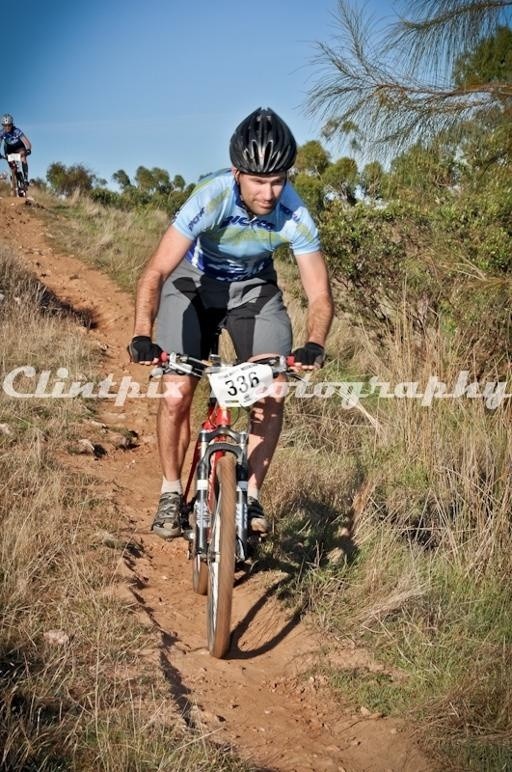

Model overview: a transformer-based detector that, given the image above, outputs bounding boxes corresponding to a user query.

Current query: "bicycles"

[150,352,328,659]
[0,149,32,199]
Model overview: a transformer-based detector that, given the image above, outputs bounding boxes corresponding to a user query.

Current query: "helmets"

[229,107,297,177]
[1,114,13,126]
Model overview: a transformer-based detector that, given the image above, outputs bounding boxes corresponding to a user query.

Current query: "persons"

[0,113,33,196]
[129,107,334,541]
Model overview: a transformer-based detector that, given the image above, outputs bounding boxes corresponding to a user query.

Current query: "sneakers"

[246,495,268,533]
[152,484,183,539]
